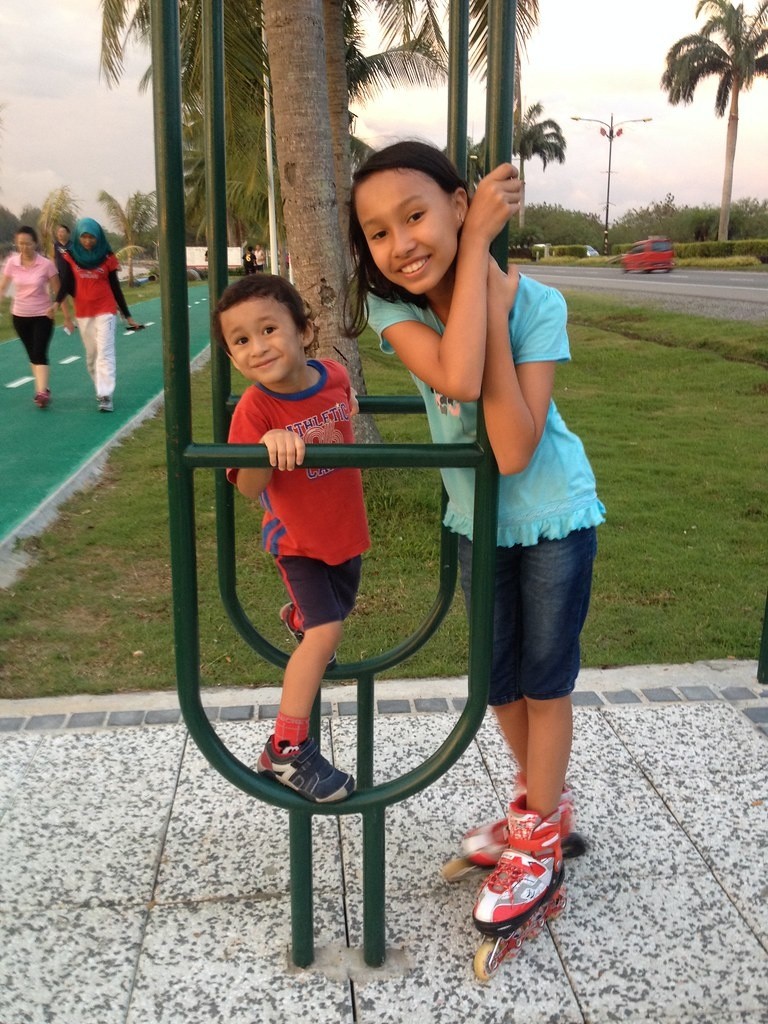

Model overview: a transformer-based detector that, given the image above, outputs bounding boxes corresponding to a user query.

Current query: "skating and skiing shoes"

[472,794,569,981]
[441,773,587,884]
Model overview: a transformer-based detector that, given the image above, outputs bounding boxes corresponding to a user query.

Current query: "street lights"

[571,113,652,256]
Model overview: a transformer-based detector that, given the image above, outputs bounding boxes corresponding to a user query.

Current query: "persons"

[212,274,371,802]
[242,246,257,275]
[254,243,266,275]
[286,253,289,269]
[336,141,606,979]
[0,226,74,408]
[46,218,140,412]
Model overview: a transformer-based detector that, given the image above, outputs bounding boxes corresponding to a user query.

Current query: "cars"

[586,246,599,257]
[621,239,676,274]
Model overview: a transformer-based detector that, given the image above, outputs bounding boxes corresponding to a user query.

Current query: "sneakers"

[96,395,101,402]
[98,396,113,411]
[257,734,355,804]
[279,602,337,672]
[33,388,50,408]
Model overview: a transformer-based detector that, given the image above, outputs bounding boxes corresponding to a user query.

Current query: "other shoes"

[64,321,74,334]
